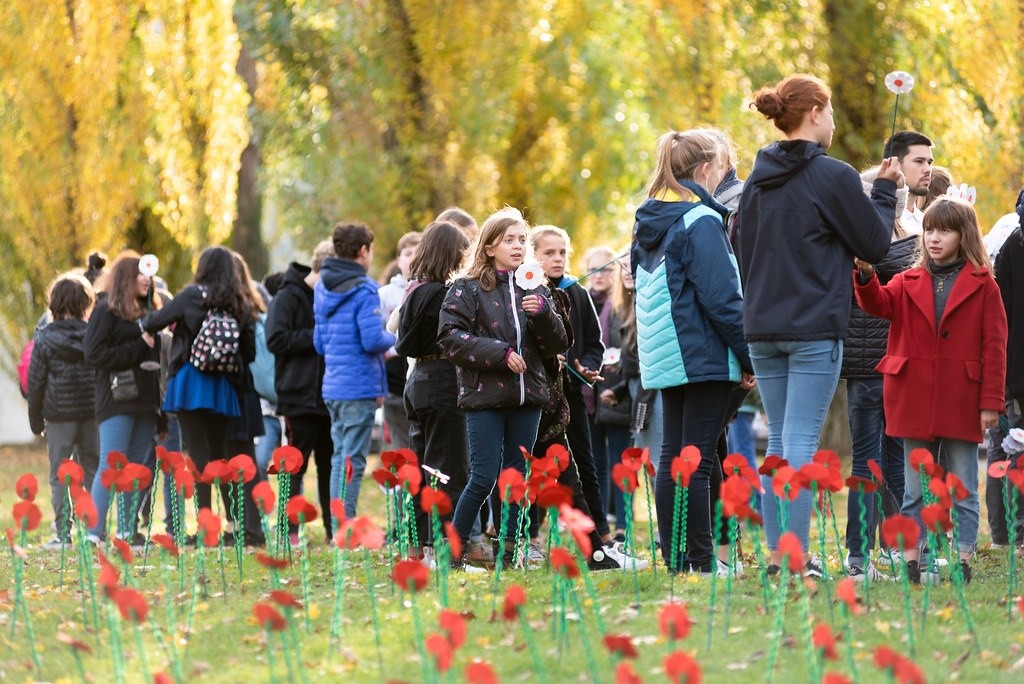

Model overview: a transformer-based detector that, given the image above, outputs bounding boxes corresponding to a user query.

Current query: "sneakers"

[919,556,948,571]
[587,546,649,573]
[846,562,890,582]
[766,565,785,578]
[878,547,909,564]
[894,565,940,585]
[790,556,833,582]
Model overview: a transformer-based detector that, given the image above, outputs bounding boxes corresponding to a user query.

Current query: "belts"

[414,353,452,363]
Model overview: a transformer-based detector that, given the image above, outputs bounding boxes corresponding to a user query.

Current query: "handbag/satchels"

[110,369,139,401]
[536,375,570,444]
[594,383,632,428]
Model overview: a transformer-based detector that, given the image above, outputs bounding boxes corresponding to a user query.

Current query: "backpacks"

[189,283,242,372]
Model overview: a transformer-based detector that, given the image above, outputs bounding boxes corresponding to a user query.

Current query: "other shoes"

[76,536,100,547]
[116,530,153,553]
[44,535,72,549]
[948,558,972,584]
[904,560,921,585]
[171,515,743,578]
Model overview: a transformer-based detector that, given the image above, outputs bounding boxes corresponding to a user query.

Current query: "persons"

[313,221,397,540]
[728,79,905,585]
[142,246,263,550]
[378,208,648,576]
[264,238,340,546]
[250,281,282,475]
[29,250,175,549]
[629,128,1024,583]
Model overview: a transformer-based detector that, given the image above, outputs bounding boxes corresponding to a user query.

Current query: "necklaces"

[933,270,958,293]
